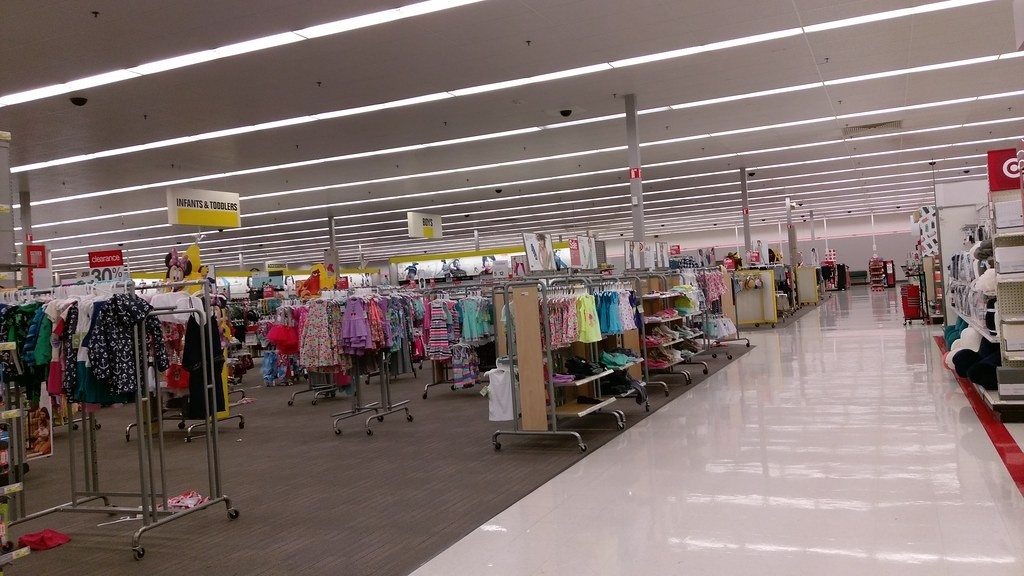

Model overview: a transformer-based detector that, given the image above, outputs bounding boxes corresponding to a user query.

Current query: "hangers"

[0,265,788,377]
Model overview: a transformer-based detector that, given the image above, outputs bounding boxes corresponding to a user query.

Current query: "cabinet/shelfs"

[491,274,669,452]
[489,285,516,357]
[631,272,719,393]
[900,148,1024,423]
[820,260,852,291]
[868,260,886,290]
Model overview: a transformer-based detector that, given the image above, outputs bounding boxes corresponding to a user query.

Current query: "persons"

[198,266,215,293]
[436,260,451,277]
[698,248,714,267]
[581,238,594,268]
[360,273,370,287]
[536,234,552,270]
[31,407,51,454]
[349,275,352,285]
[448,259,467,276]
[629,241,634,269]
[812,248,817,265]
[284,276,295,290]
[659,244,665,267]
[479,256,496,275]
[404,261,418,280]
[262,279,274,288]
[755,240,765,265]
[638,244,644,270]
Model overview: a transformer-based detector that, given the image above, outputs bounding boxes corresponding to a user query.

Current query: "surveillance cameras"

[620,203,901,239]
[219,249,222,252]
[963,171,969,174]
[118,244,124,247]
[177,242,182,244]
[929,162,936,165]
[495,189,502,193]
[324,249,328,251]
[219,229,224,232]
[71,97,88,106]
[747,173,756,176]
[465,215,469,217]
[560,110,572,117]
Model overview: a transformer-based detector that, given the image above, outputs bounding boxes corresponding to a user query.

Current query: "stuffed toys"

[161,247,192,291]
[943,296,1000,390]
